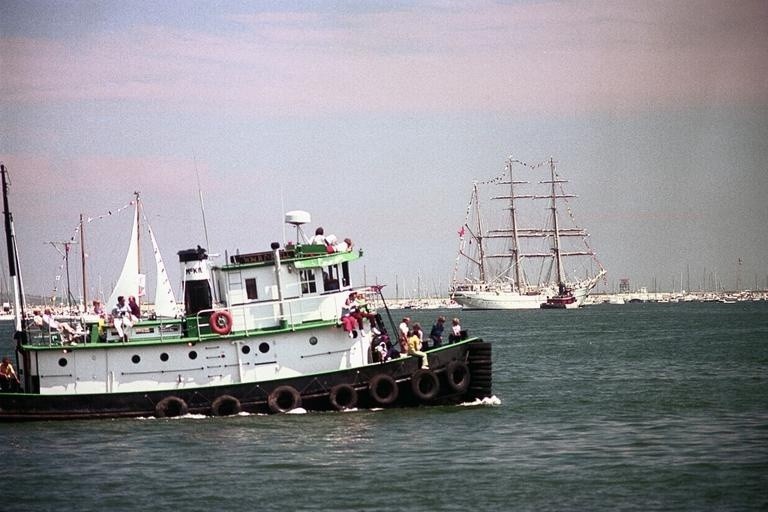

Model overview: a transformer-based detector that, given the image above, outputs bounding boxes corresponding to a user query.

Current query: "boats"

[1,160,492,418]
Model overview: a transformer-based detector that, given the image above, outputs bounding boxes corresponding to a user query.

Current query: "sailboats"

[448,156,607,309]
[657,264,768,303]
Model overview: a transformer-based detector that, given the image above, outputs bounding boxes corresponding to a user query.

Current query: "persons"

[449,316,462,344]
[312,227,325,246]
[32,308,79,347]
[90,299,107,343]
[411,322,423,352]
[1,355,22,386]
[398,315,412,355]
[341,291,381,339]
[324,239,353,254]
[43,306,77,347]
[126,296,141,328]
[111,294,132,342]
[407,329,430,372]
[0,364,19,392]
[383,340,401,363]
[428,315,446,347]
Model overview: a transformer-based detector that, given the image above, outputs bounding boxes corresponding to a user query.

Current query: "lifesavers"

[210,311,233,334]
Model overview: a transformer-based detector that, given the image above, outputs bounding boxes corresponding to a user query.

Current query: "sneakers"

[371,327,381,335]
[348,333,353,339]
[360,330,366,337]
[421,365,430,370]
